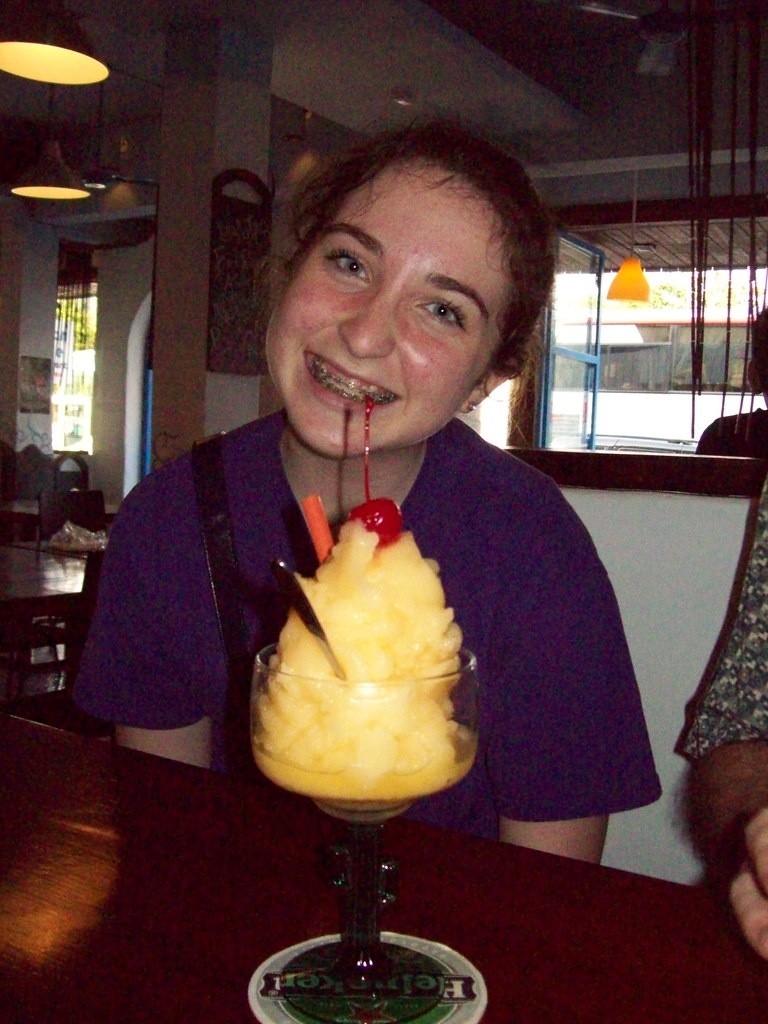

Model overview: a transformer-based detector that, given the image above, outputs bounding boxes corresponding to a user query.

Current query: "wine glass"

[242,644,481,1024]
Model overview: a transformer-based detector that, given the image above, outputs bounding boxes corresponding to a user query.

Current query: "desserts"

[251,395,474,802]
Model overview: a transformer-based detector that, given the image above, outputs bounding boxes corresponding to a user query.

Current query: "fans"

[582,0,766,78]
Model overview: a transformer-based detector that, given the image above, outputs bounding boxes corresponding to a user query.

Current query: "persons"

[81,108,666,866]
[700,304,768,459]
[677,475,768,966]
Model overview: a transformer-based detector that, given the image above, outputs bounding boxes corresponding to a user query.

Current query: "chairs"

[0,441,119,687]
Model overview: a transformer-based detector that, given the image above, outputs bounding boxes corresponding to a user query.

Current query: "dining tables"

[1,717,768,1024]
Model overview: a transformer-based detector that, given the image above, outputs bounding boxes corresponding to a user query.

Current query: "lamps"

[11,82,90,200]
[608,171,651,302]
[1,1,109,85]
